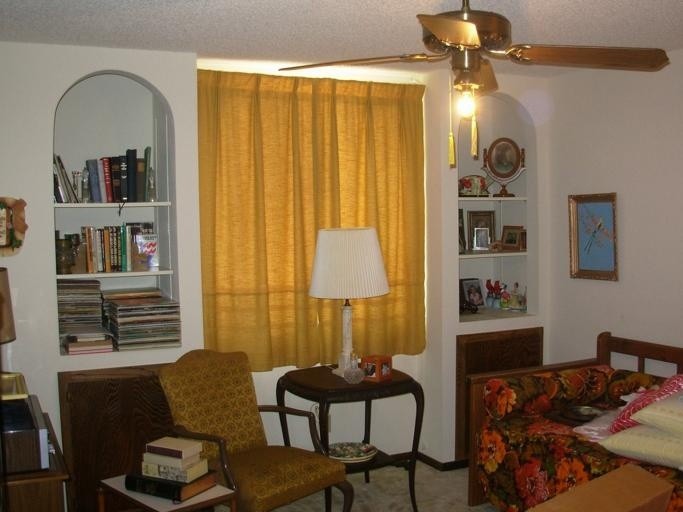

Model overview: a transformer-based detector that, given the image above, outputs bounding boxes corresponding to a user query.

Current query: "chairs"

[156,347,354,512]
[524,462,674,512]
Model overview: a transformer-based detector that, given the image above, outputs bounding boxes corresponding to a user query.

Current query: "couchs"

[463,330,682,512]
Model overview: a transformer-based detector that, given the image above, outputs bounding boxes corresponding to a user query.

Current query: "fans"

[276,1,671,95]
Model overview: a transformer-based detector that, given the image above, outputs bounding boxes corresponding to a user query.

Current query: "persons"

[469,284,483,305]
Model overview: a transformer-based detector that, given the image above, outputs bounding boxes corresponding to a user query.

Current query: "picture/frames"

[500,224,523,251]
[567,191,620,282]
[466,210,496,253]
[360,354,393,383]
[1,195,28,259]
[482,137,527,197]
[462,277,488,309]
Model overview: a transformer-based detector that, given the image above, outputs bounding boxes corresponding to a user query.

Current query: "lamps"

[447,1,486,168]
[306,225,391,377]
[0,267,17,345]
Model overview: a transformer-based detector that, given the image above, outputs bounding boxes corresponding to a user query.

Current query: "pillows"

[609,372,683,432]
[629,389,683,431]
[599,425,683,471]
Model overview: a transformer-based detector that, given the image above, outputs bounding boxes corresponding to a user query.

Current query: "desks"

[0,371,72,512]
[99,472,235,511]
[274,366,425,512]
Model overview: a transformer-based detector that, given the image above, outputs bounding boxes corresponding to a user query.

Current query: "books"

[124,436,218,502]
[53,145,182,355]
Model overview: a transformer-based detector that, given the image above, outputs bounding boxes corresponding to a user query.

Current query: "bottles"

[343,351,366,385]
[486,293,501,309]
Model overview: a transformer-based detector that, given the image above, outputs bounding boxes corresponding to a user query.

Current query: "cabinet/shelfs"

[456,192,547,465]
[56,200,197,511]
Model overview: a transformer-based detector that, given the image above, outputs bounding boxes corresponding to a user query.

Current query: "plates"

[329,442,378,464]
